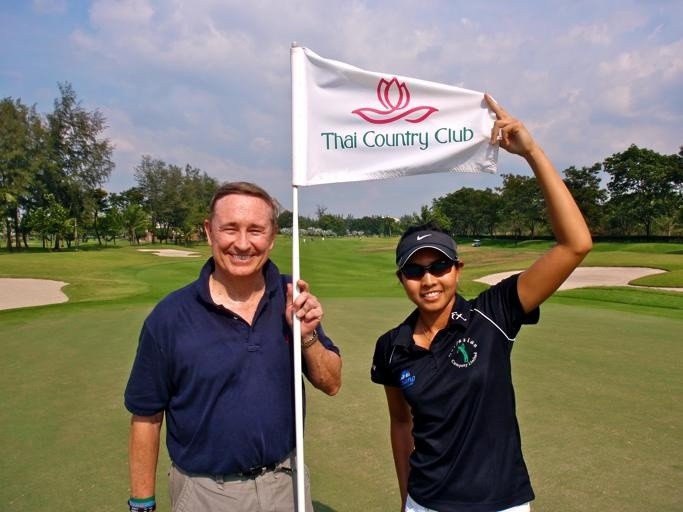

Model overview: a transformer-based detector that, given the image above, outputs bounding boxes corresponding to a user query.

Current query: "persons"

[121,181,341,512]
[370,92,591,512]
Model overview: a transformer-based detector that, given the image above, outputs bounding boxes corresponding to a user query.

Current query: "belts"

[215,463,276,482]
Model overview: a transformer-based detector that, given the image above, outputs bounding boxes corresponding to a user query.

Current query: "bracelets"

[127,499,154,507]
[129,494,154,503]
[129,505,155,512]
[302,330,318,348]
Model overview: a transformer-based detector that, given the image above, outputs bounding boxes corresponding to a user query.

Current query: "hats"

[396,230,457,269]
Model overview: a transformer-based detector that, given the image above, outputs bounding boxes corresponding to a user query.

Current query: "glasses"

[401,259,453,279]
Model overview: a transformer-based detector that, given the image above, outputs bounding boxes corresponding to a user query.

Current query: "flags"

[289,46,497,186]
